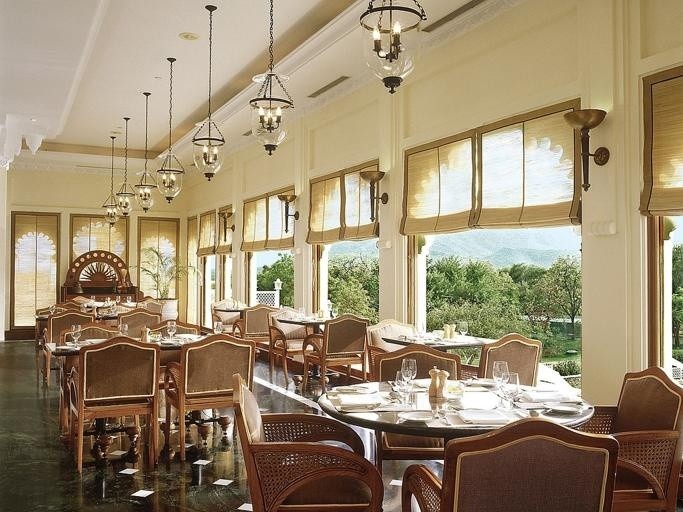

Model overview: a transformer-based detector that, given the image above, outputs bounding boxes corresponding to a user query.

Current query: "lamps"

[356,168,390,222]
[101,52,184,228]
[563,107,613,191]
[216,211,235,243]
[245,0,295,158]
[358,0,429,94]
[191,1,226,184]
[277,192,302,234]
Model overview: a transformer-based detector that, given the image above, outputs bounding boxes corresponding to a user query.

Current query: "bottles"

[441,323,455,340]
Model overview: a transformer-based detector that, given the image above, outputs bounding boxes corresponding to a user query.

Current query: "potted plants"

[129,244,198,320]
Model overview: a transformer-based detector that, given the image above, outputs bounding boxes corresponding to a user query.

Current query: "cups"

[415,321,424,338]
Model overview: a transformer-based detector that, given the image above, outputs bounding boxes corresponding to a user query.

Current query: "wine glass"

[48,305,55,315]
[395,360,519,408]
[70,320,222,349]
[459,322,467,338]
[90,295,132,317]
[287,302,337,320]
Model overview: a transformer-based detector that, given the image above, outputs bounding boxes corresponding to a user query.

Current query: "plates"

[332,378,584,423]
[55,333,204,349]
